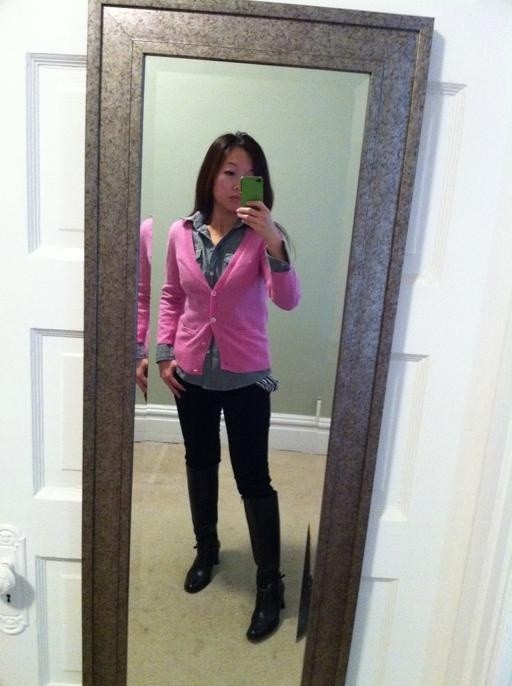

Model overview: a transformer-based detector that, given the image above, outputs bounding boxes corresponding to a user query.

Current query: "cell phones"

[240,176,263,207]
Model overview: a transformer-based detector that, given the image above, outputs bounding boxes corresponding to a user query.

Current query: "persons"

[136,215,155,404]
[158,131,303,645]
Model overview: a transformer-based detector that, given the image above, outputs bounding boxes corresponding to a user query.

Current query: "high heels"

[246,581,286,642]
[184,542,221,593]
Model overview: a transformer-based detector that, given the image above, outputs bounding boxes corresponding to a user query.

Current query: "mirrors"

[81,0,437,684]
